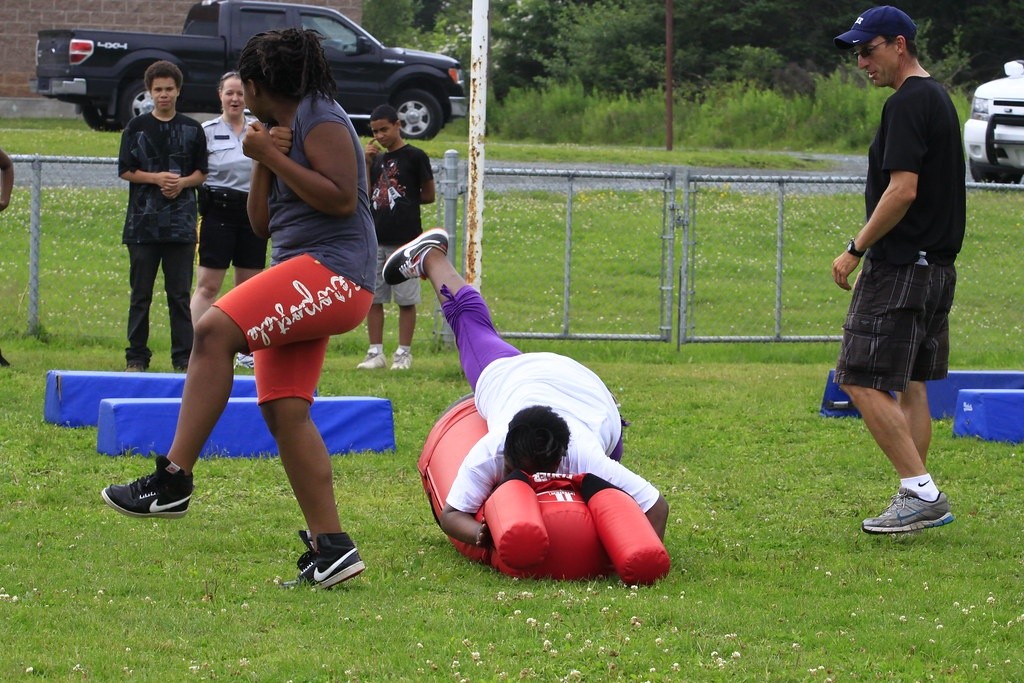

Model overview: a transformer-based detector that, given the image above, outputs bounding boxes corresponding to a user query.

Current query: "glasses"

[854,39,888,56]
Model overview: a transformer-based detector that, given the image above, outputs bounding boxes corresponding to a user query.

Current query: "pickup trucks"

[33,0,466,142]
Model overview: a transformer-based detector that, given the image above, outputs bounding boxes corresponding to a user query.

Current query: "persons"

[191,72,266,369]
[355,104,436,369]
[118,60,210,374]
[0,149,14,369]
[831,5,966,534]
[102,28,380,590]
[381,227,669,548]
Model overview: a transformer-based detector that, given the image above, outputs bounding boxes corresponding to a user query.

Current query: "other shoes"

[174,365,188,373]
[123,363,145,373]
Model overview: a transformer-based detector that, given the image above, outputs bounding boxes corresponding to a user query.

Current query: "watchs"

[848,240,866,258]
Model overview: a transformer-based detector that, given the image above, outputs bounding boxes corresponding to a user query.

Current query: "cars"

[962,60,1024,186]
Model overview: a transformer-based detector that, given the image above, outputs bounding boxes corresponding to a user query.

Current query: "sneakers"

[102,456,194,518]
[390,349,413,371]
[357,347,388,370]
[280,529,365,591]
[381,227,448,286]
[862,487,954,535]
[233,354,254,370]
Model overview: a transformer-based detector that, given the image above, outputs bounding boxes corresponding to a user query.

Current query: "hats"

[833,5,918,50]
